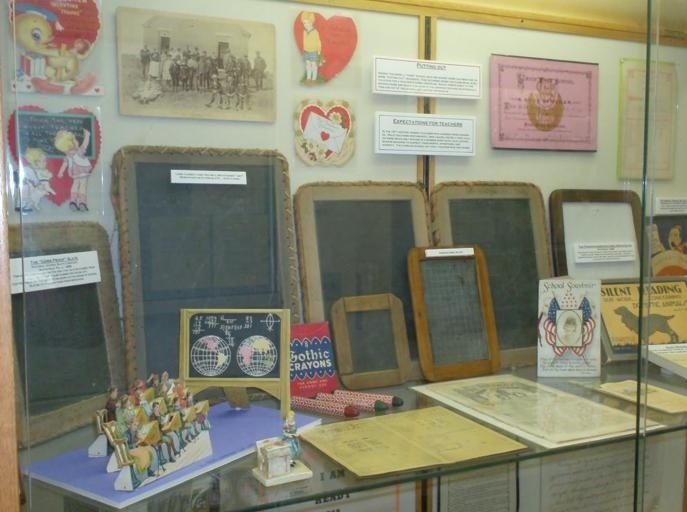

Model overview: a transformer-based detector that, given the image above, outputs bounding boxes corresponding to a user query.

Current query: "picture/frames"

[405,244,501,383]
[113,5,279,124]
[546,186,643,280]
[426,178,556,370]
[108,142,303,409]
[405,371,670,452]
[177,306,293,420]
[329,292,414,391]
[598,274,687,366]
[292,179,436,383]
[10,219,129,452]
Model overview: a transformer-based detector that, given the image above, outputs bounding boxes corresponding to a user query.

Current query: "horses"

[204,65,253,112]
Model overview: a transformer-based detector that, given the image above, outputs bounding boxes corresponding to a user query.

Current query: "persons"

[142,41,264,112]
